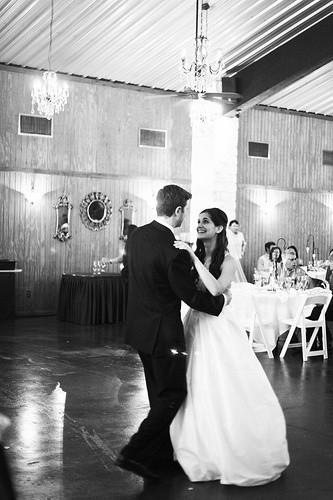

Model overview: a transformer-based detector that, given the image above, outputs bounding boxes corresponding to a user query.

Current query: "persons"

[173,208,290,487]
[102,224,137,264]
[257,240,333,290]
[114,185,232,500]
[226,219,247,260]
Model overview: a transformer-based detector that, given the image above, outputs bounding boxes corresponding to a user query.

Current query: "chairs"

[242,295,275,358]
[279,295,333,361]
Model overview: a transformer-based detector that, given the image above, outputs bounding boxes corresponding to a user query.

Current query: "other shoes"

[115,454,161,481]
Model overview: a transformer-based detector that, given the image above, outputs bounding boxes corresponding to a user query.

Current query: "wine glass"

[308,260,330,271]
[255,272,307,290]
[93,260,106,275]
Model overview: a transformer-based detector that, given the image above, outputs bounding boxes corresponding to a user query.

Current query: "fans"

[141,0,243,107]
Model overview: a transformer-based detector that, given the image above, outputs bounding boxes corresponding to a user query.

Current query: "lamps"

[180,0,223,78]
[31,0,69,120]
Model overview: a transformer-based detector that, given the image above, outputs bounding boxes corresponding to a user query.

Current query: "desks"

[232,282,332,353]
[56,273,128,326]
